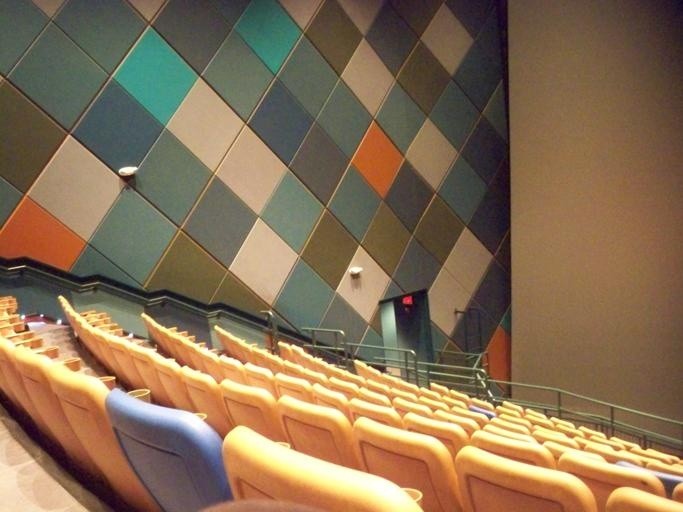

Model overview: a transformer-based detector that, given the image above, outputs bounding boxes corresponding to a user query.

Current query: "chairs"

[449,389,469,404]
[430,382,449,396]
[366,366,381,382]
[454,445,598,512]
[244,362,274,398]
[483,423,540,443]
[645,461,683,476]
[353,359,367,379]
[154,323,167,348]
[301,353,312,369]
[141,313,152,333]
[579,426,607,440]
[107,336,146,389]
[532,424,566,437]
[215,326,230,352]
[418,395,450,412]
[128,343,174,407]
[329,376,358,400]
[58,296,96,329]
[525,409,548,421]
[153,356,197,413]
[496,406,521,418]
[63,303,107,346]
[590,436,625,451]
[433,408,480,438]
[532,429,580,449]
[200,350,220,383]
[171,334,193,367]
[380,373,396,387]
[402,412,469,460]
[366,379,390,397]
[274,373,311,403]
[228,334,245,358]
[550,417,575,429]
[312,359,327,374]
[399,381,420,397]
[81,323,114,373]
[311,382,350,425]
[442,395,468,409]
[503,401,524,413]
[284,360,304,378]
[672,482,683,502]
[490,416,532,436]
[498,413,532,431]
[14,343,107,482]
[49,359,164,512]
[557,451,667,512]
[94,329,131,385]
[251,347,268,366]
[541,441,608,463]
[574,436,613,450]
[239,342,253,361]
[525,414,555,431]
[69,312,97,357]
[278,341,290,359]
[327,364,342,377]
[556,424,585,437]
[615,460,683,498]
[351,416,463,512]
[303,368,328,385]
[266,353,283,372]
[468,404,495,420]
[344,371,364,388]
[218,378,286,443]
[533,425,567,437]
[630,447,672,465]
[604,485,683,512]
[391,397,434,417]
[646,448,680,464]
[162,328,183,362]
[471,397,495,410]
[105,385,232,512]
[291,345,303,362]
[0,296,59,348]
[348,398,402,428]
[222,425,426,512]
[358,387,392,406]
[180,364,233,438]
[390,389,417,404]
[583,444,643,466]
[220,356,242,384]
[183,342,205,370]
[469,430,557,473]
[419,386,441,401]
[610,437,641,451]
[275,395,360,471]
[450,406,489,428]
